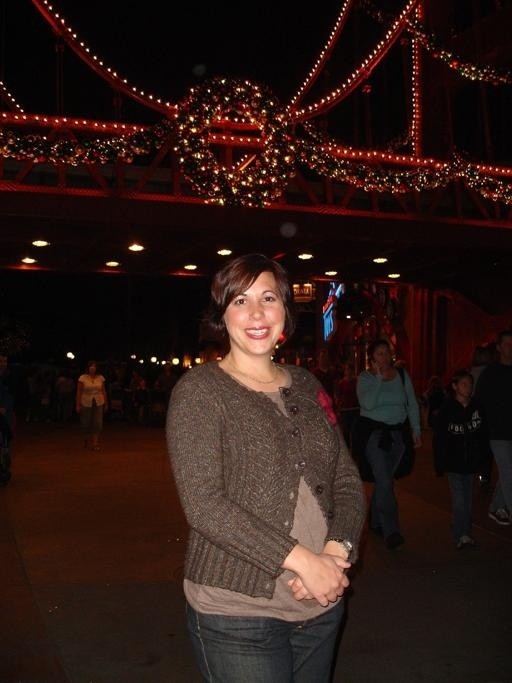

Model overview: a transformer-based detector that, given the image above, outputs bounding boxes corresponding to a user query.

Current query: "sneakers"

[456,535,481,559]
[487,508,512,526]
[367,527,407,549]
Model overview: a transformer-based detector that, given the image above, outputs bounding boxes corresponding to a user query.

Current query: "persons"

[25,353,180,428]
[334,362,360,447]
[432,370,494,550]
[475,327,512,525]
[354,335,423,552]
[309,346,343,400]
[161,251,369,683]
[0,352,16,453]
[416,365,458,479]
[74,359,110,451]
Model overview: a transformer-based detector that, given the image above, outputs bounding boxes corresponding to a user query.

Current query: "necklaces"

[227,358,280,384]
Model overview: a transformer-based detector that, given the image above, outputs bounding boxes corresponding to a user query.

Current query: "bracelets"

[337,538,354,558]
